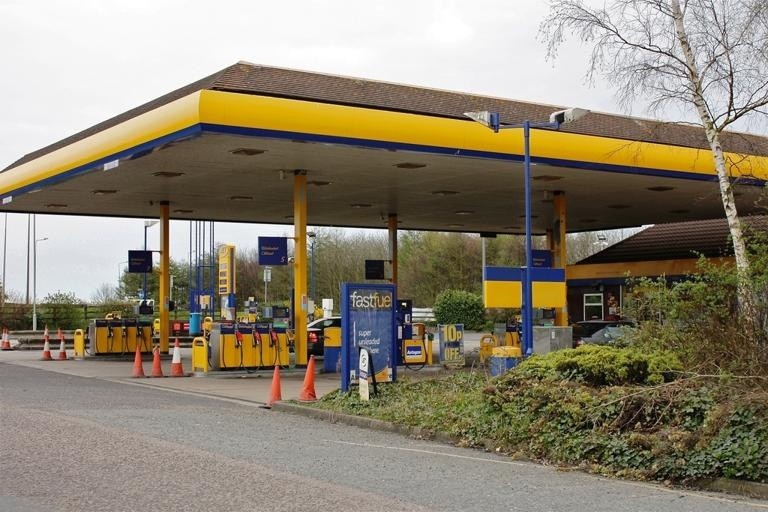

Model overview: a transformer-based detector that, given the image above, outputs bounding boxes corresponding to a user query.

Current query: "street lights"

[33,237,49,330]
[305,230,315,322]
[143,218,157,317]
[460,105,592,365]
[289,255,296,325]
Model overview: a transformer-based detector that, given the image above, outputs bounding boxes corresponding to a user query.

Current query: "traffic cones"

[296,354,318,403]
[38,335,54,360]
[57,326,63,340]
[258,365,284,408]
[1,327,13,351]
[44,322,50,339]
[55,336,70,361]
[127,343,148,379]
[144,343,167,377]
[167,336,191,377]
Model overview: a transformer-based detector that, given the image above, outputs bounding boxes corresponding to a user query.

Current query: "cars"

[286,315,341,360]
[568,320,642,349]
